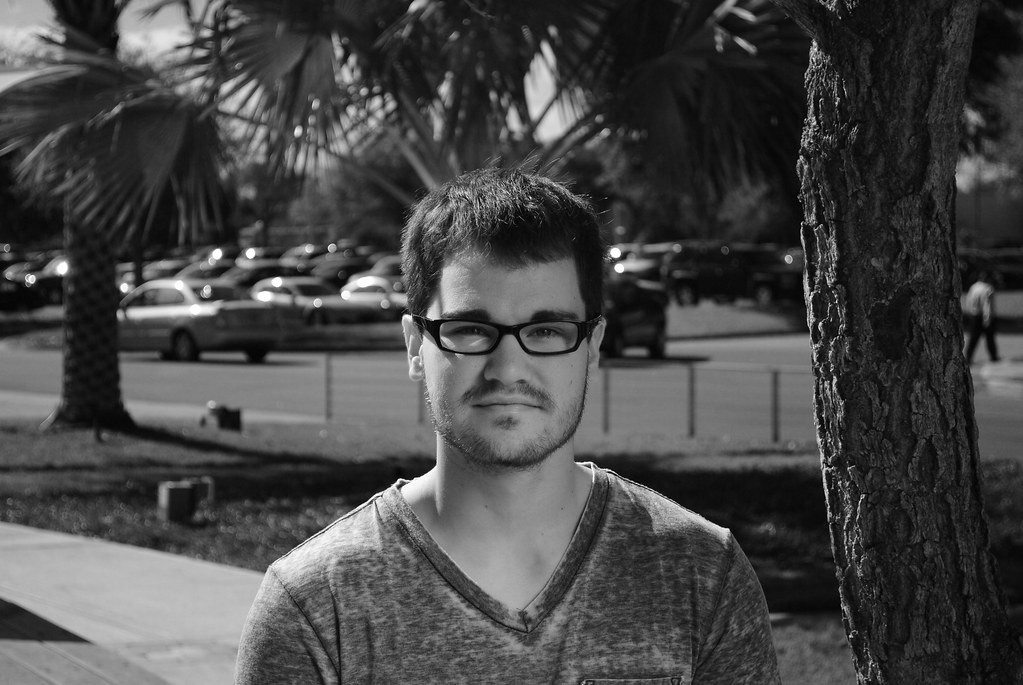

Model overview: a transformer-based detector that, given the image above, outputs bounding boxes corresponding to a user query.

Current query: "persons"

[235,167,785,685]
[964,267,1002,364]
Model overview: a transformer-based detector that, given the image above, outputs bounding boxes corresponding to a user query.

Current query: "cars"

[1,233,807,360]
[121,274,282,365]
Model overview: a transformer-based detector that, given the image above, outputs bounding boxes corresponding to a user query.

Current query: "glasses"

[410,311,603,356]
